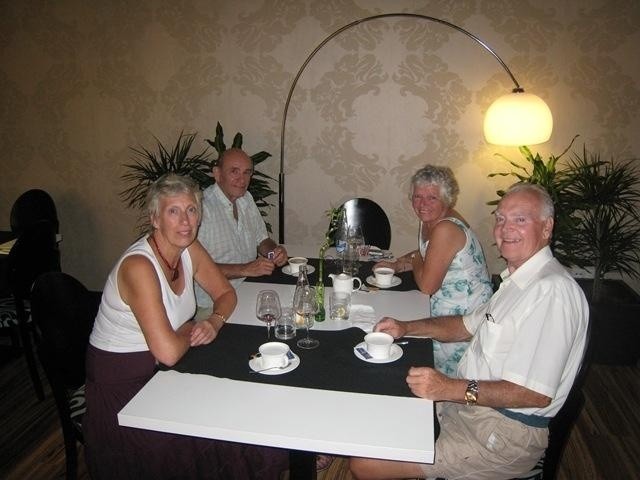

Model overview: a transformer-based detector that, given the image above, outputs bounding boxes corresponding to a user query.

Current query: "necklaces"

[150,233,181,281]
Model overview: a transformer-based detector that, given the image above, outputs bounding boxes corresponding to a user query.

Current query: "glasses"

[487,313,495,324]
[407,194,437,201]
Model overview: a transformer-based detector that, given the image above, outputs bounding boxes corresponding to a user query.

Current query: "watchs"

[464,378,479,406]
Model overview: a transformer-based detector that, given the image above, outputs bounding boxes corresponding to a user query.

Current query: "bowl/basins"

[257,342,291,371]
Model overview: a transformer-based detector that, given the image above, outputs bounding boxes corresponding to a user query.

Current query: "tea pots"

[328,272,361,298]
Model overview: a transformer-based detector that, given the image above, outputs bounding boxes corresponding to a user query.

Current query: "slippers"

[266,456,331,472]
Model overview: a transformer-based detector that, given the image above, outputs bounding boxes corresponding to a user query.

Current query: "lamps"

[278,14,554,249]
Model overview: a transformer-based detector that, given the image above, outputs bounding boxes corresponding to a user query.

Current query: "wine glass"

[292,285,321,350]
[348,223,365,256]
[255,288,282,344]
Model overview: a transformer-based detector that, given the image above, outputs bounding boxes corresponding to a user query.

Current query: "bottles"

[292,264,315,327]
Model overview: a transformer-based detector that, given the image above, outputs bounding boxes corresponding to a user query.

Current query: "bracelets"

[409,251,420,264]
[213,312,226,324]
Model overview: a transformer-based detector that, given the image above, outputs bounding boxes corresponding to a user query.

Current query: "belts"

[494,405,551,428]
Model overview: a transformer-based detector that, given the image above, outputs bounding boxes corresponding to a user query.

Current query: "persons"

[372,164,494,379]
[347,181,591,480]
[83,173,335,479]
[192,147,288,321]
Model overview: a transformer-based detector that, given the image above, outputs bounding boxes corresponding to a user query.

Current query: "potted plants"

[563,149,640,362]
[314,207,344,322]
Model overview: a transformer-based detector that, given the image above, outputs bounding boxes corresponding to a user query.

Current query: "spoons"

[245,363,292,374]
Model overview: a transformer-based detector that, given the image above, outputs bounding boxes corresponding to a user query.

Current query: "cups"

[274,307,297,341]
[327,291,351,321]
[343,247,360,275]
[363,332,395,359]
[288,257,308,273]
[374,267,395,285]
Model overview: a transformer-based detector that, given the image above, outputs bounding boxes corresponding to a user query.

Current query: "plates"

[249,350,301,377]
[366,275,402,288]
[353,340,404,364]
[282,264,315,277]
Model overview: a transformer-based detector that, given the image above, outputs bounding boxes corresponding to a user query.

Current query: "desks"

[0,231,64,272]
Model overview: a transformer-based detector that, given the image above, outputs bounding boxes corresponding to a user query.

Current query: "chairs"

[327,197,391,250]
[0,189,61,235]
[28,271,87,479]
[514,345,594,480]
[490,272,505,295]
[0,230,64,402]
